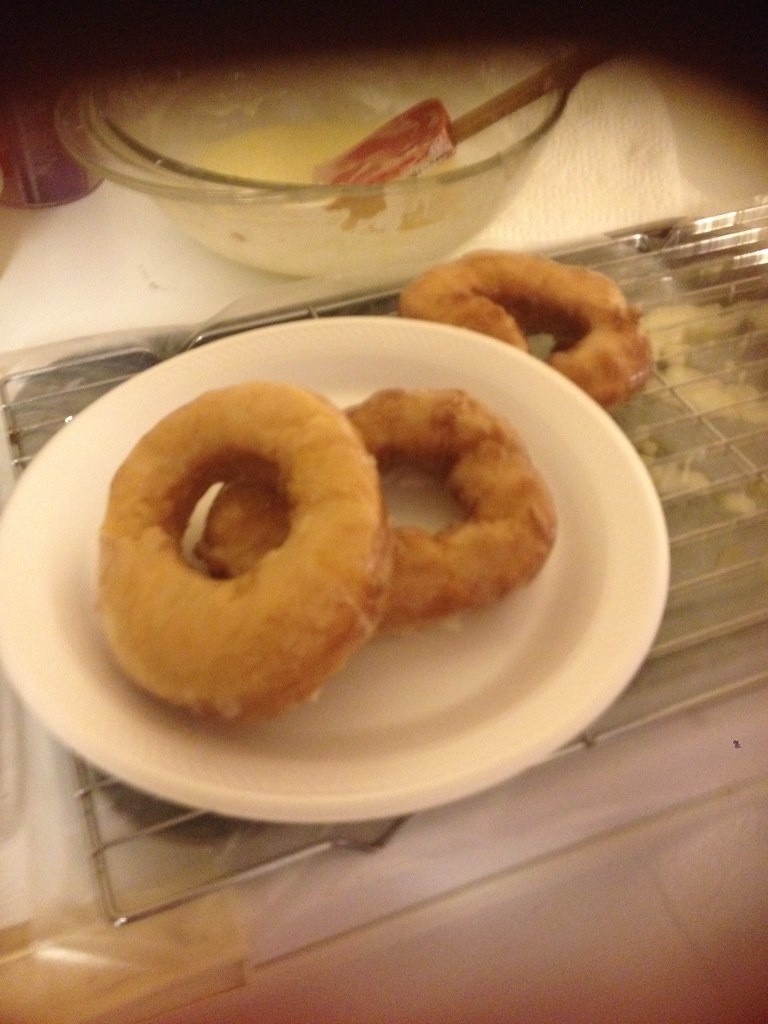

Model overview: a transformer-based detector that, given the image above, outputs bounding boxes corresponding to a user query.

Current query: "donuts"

[190,386,559,635]
[395,247,654,413]
[97,376,395,723]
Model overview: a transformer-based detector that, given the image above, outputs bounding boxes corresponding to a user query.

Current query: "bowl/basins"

[51,36,578,283]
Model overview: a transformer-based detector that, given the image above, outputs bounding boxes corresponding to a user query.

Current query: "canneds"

[0,0,107,208]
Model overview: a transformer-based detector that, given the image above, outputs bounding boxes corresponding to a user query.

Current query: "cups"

[0,39,108,216]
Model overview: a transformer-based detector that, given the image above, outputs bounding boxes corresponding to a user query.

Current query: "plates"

[0,315,675,825]
